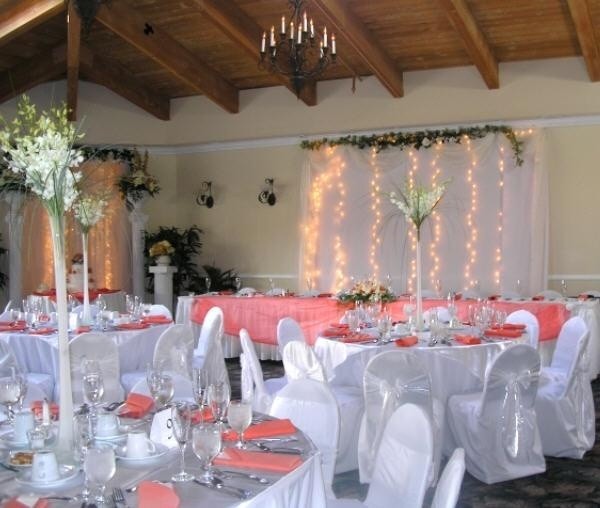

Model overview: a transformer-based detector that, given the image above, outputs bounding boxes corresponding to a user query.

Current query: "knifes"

[251,441,302,456]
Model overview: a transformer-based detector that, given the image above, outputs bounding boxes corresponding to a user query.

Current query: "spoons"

[213,476,248,496]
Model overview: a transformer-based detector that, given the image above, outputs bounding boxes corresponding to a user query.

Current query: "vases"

[83,232,89,320]
[50,216,74,456]
[155,255,172,266]
[415,241,423,330]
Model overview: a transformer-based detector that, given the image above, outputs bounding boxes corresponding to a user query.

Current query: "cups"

[560,279,569,301]
[432,279,443,296]
[31,449,60,483]
[124,429,156,457]
[514,279,524,299]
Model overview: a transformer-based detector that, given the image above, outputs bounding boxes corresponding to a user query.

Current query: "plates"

[7,451,33,468]
[116,443,168,463]
[17,465,79,487]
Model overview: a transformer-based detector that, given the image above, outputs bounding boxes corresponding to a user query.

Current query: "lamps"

[258,0,336,100]
[258,178,276,206]
[196,181,213,209]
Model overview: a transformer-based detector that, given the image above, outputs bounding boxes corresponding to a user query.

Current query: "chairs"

[0,288,600,508]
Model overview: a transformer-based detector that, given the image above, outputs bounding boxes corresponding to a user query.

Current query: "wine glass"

[0,365,22,417]
[306,278,314,300]
[191,368,209,412]
[235,278,242,297]
[206,380,230,434]
[170,401,195,483]
[192,421,223,485]
[145,358,175,407]
[205,277,212,294]
[9,293,153,332]
[269,278,277,296]
[83,359,104,416]
[342,291,507,344]
[226,398,253,450]
[84,439,117,508]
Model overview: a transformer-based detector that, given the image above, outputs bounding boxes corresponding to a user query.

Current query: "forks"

[193,479,245,500]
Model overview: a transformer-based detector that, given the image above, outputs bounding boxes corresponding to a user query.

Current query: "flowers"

[354,171,454,241]
[298,125,523,168]
[148,240,175,256]
[0,78,162,255]
